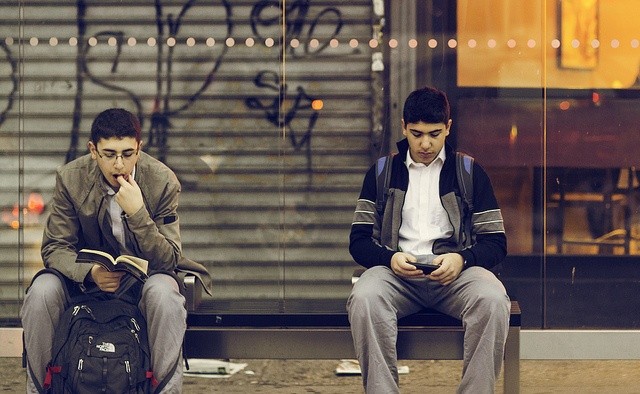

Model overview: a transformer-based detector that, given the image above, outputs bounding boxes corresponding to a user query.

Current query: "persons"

[17,105,214,393]
[344,85,512,394]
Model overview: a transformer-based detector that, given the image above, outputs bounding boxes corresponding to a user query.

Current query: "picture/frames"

[555,0,600,70]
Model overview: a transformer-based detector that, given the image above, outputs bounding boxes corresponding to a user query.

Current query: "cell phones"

[406,258,448,281]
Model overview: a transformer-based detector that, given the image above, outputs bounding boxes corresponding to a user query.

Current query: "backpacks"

[21,268,190,393]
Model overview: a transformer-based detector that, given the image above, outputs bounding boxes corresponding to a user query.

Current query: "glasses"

[94,143,140,161]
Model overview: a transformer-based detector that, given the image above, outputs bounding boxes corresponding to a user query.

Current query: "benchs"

[22,298,522,394]
